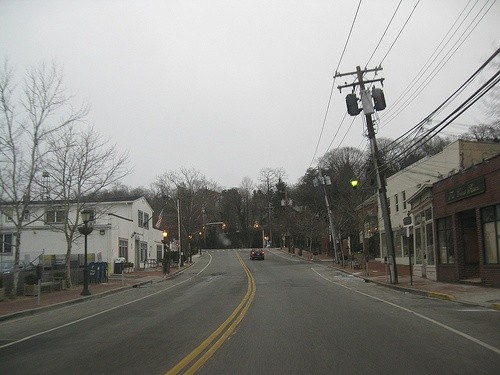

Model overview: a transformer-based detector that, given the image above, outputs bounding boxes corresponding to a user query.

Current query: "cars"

[250,248,265,260]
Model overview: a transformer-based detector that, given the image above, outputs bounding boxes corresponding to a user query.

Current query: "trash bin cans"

[115,263,123,274]
[163,261,170,272]
[88,262,108,282]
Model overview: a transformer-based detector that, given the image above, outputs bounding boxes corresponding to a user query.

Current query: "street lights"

[349,176,398,284]
[78,210,94,295]
[199,231,202,255]
[162,229,168,273]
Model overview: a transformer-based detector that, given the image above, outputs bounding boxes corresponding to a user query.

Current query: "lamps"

[437,152,498,188]
[100,230,105,235]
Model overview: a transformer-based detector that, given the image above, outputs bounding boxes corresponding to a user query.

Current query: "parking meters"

[384,256,388,282]
[121,261,124,285]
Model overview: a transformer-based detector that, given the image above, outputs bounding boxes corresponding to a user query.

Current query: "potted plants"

[124,262,135,273]
[23,271,40,296]
[52,270,68,289]
